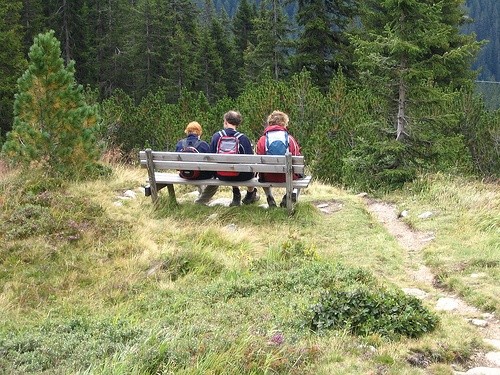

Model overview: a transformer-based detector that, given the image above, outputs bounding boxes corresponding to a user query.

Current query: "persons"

[210,110,260,207]
[175,121,220,207]
[255,110,303,208]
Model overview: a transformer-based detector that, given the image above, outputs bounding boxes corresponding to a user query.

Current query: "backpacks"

[179,139,203,180]
[216,129,244,177]
[265,130,290,155]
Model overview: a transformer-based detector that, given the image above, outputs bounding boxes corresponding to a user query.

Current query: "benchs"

[139,147,312,218]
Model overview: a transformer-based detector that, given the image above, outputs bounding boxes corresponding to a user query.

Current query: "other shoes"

[242,188,261,205]
[229,193,241,207]
[194,198,208,206]
[280,194,287,208]
[266,195,277,208]
